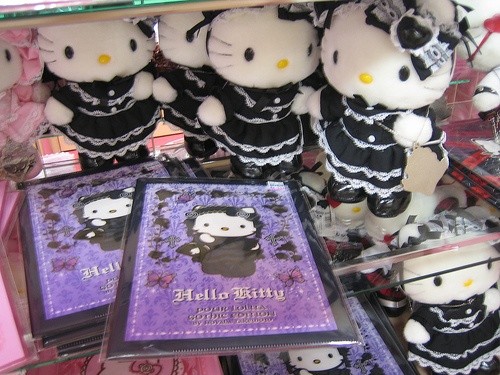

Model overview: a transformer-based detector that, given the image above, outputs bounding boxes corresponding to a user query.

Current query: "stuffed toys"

[454,0,500,122]
[194,4,321,179]
[0,25,51,182]
[306,0,460,217]
[396,206,499,375]
[153,10,222,159]
[36,17,162,159]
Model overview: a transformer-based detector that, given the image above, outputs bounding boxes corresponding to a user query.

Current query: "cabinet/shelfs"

[0,0,500,375]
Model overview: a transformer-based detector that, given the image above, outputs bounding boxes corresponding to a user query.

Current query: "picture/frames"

[14,153,214,358]
[106,179,358,361]
[218,272,420,375]
